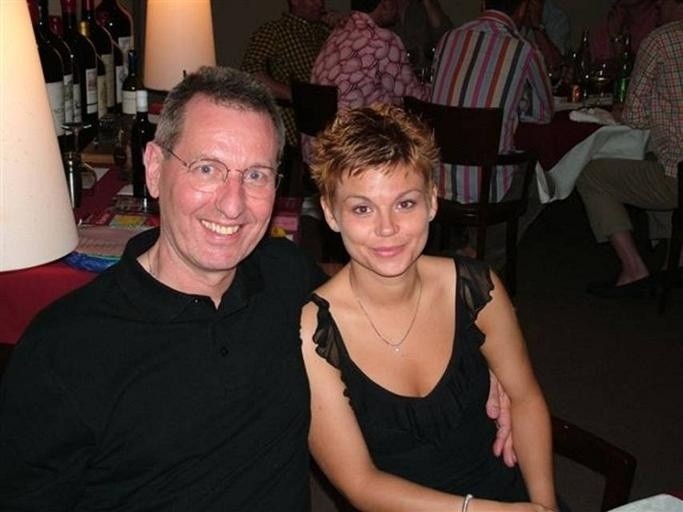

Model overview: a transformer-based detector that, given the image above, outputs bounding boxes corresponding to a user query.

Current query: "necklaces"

[349,266,422,352]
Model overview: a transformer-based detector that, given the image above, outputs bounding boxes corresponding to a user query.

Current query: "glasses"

[156,142,282,201]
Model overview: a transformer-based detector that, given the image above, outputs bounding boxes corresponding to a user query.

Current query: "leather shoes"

[588,274,650,297]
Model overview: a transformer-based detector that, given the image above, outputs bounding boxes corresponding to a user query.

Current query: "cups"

[415,64,432,86]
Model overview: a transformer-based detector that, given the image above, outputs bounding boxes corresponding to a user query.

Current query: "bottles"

[621,33,636,74]
[575,33,592,76]
[33,0,157,215]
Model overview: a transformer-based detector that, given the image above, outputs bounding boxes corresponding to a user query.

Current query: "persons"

[0,66,519,512]
[240,1,683,301]
[298,102,561,512]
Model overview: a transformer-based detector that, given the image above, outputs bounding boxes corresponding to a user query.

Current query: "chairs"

[304,406,636,511]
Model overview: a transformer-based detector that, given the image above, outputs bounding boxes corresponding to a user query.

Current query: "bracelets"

[462,493,473,512]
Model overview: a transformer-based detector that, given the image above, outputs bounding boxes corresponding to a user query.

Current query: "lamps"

[0,0,80,273]
[144,1,217,98]
